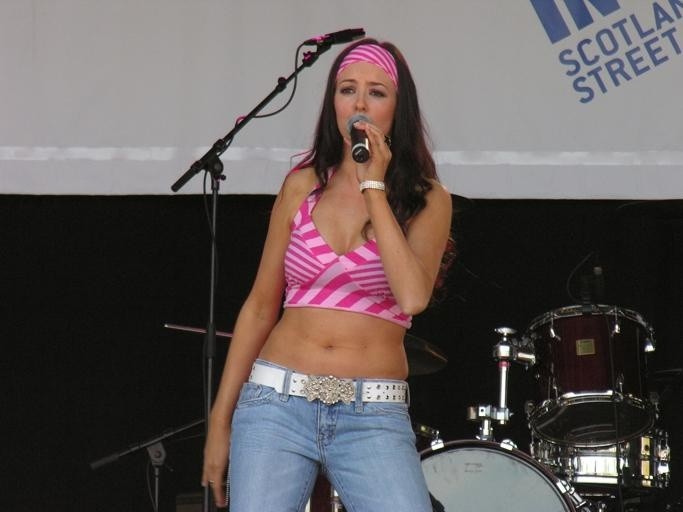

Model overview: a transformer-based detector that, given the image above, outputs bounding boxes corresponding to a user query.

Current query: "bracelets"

[358,180,384,193]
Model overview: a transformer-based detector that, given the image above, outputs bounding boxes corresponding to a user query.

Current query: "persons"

[200,36,454,512]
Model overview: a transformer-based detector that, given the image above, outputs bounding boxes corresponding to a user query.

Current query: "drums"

[531,424,673,499]
[418,436,597,512]
[524,303,659,443]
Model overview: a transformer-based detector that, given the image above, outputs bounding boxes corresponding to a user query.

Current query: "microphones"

[346,114,371,163]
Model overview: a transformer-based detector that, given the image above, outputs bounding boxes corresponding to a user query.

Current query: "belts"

[249,360,408,407]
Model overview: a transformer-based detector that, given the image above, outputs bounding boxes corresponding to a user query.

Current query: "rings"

[207,479,214,486]
[382,134,393,148]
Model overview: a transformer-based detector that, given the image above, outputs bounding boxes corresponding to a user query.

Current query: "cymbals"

[403,335,448,377]
